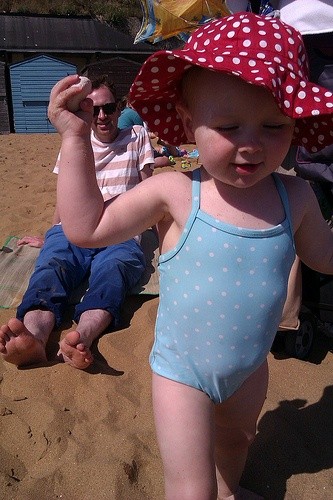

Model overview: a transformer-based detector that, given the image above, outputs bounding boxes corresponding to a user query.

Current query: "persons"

[117,91,171,169]
[0,75,156,375]
[157,136,180,156]
[47,12,333,500]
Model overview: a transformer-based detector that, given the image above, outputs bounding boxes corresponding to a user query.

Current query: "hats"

[127,11,333,153]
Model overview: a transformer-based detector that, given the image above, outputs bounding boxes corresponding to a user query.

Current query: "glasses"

[92,101,117,116]
